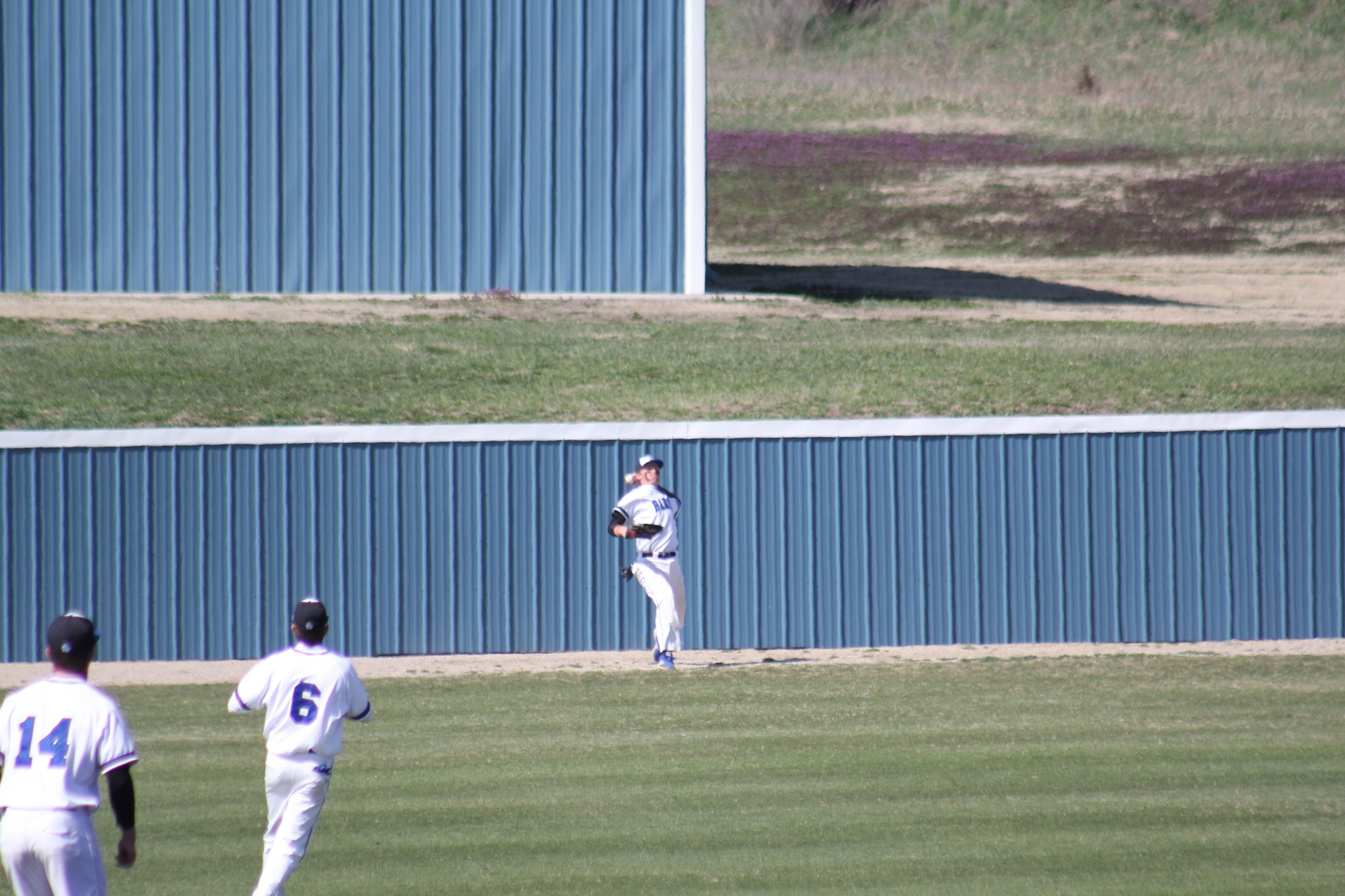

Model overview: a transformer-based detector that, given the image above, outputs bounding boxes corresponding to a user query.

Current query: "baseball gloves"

[625,524,664,540]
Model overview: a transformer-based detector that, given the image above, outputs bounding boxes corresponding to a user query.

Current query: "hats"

[46,612,100,655]
[635,455,663,470]
[291,599,328,630]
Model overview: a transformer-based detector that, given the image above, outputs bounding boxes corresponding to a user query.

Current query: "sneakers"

[658,651,674,670]
[651,629,663,663]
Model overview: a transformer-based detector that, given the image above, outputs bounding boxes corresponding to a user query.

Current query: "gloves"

[314,764,331,776]
[620,567,633,581]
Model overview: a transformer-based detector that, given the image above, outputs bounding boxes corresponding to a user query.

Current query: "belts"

[643,552,674,558]
[1,806,76,812]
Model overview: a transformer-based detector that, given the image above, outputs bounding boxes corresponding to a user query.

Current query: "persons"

[607,455,686,670]
[227,598,373,896]
[0,611,139,896]
